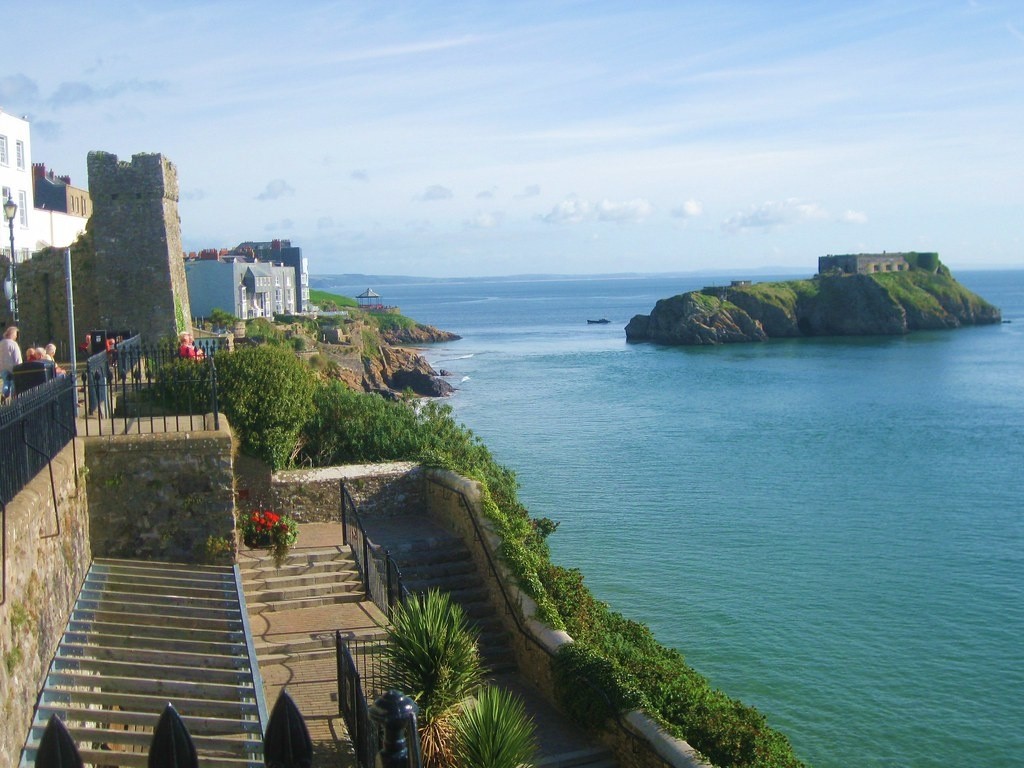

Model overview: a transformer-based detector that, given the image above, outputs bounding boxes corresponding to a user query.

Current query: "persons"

[26,343,66,376]
[0,325,23,398]
[179,331,208,361]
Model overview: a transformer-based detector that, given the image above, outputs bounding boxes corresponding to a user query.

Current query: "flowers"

[237,511,300,548]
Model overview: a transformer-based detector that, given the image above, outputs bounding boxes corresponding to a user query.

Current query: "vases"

[242,533,277,549]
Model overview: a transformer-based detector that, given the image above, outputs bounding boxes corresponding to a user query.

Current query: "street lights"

[3,190,22,345]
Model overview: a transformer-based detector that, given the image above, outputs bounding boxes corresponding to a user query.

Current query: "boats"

[586,317,611,325]
